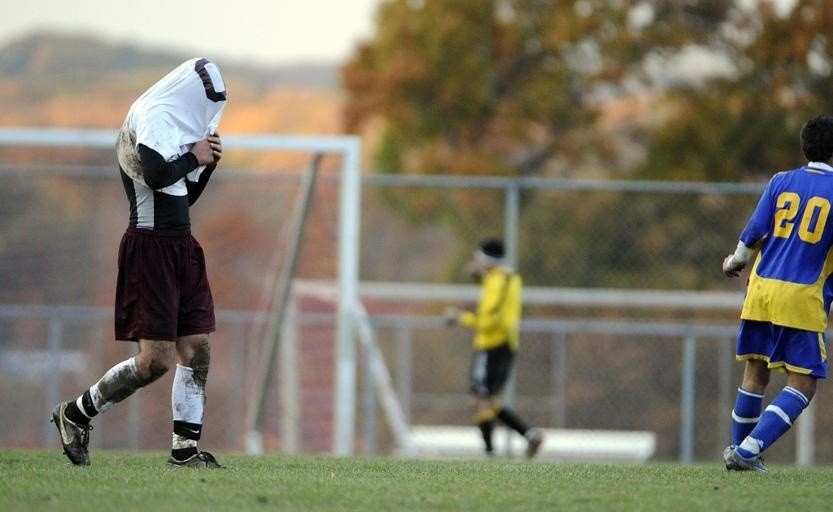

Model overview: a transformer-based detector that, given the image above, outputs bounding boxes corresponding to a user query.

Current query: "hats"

[478,239,505,257]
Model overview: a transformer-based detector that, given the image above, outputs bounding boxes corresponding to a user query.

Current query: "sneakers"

[166,451,220,469]
[527,430,543,457]
[723,444,768,471]
[50,400,91,466]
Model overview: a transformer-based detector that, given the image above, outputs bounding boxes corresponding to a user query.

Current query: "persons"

[47,55,229,467]
[441,236,545,458]
[716,109,832,474]
